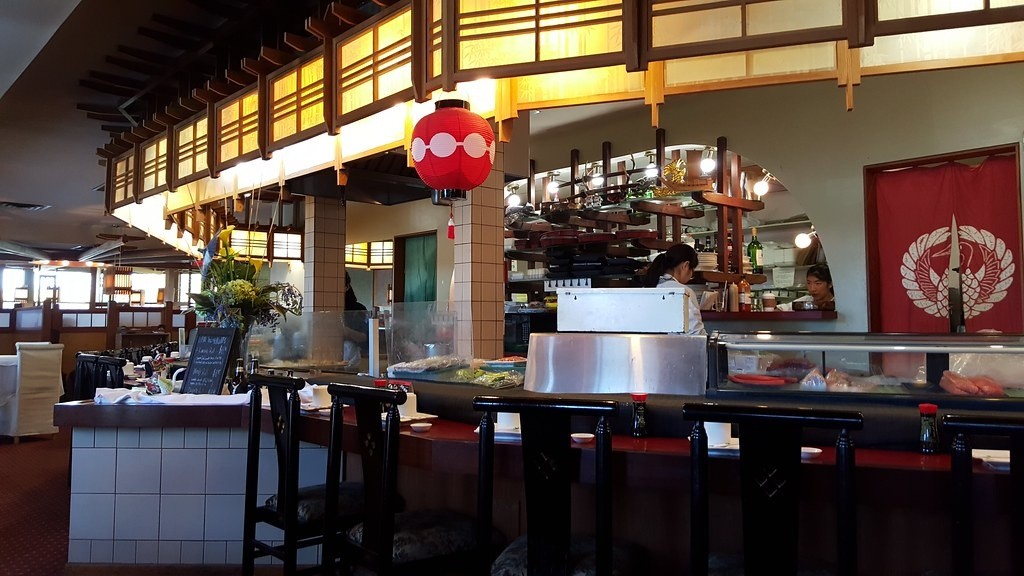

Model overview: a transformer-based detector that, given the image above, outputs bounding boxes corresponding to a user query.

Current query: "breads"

[799,368,871,392]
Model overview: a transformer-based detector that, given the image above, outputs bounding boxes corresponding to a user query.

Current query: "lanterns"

[410,99,496,240]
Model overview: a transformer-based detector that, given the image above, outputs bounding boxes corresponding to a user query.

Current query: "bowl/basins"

[410,423,432,431]
[801,447,822,459]
[319,409,330,416]
[571,434,594,443]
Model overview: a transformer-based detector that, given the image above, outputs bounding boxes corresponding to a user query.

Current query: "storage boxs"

[763,246,800,264]
[556,286,690,332]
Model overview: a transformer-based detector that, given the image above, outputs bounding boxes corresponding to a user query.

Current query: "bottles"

[373,380,414,413]
[228,358,275,395]
[729,282,739,311]
[630,393,648,438]
[746,229,763,274]
[694,231,747,265]
[919,404,940,454]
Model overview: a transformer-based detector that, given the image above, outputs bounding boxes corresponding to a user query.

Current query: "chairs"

[321,382,511,575]
[245,372,407,576]
[683,403,866,576]
[939,412,1024,576]
[69,339,177,484]
[471,394,649,576]
[0,341,64,444]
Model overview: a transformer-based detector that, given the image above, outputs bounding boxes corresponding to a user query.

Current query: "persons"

[774,264,835,311]
[343,269,369,371]
[644,243,709,335]
[158,324,171,342]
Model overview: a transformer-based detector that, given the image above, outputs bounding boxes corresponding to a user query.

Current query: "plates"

[482,360,526,367]
[697,252,718,269]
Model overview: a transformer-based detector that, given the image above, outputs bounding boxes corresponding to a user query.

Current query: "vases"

[226,328,251,383]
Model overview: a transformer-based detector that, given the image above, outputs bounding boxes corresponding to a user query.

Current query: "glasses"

[690,268,695,279]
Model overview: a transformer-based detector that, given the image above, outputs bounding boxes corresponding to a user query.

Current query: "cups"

[762,293,776,311]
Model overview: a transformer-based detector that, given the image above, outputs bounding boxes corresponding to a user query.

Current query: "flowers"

[179,224,305,378]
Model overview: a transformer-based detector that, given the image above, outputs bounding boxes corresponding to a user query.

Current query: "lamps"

[506,183,520,207]
[752,167,776,195]
[589,161,604,185]
[645,150,658,176]
[794,226,818,248]
[700,146,715,171]
[546,169,560,194]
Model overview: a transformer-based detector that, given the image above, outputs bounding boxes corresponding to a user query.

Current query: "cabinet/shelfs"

[505,135,768,303]
[665,219,827,292]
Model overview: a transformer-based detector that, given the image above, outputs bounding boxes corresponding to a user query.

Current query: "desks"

[0,354,20,390]
[115,331,171,347]
